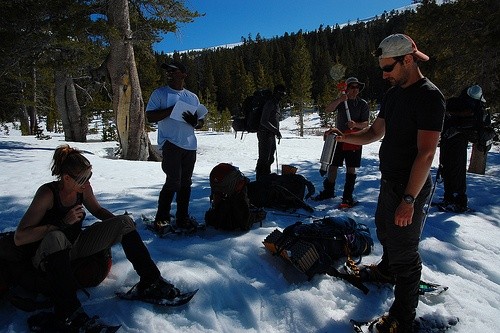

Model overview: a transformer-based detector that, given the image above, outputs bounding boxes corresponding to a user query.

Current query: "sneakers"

[137,276,179,299]
[316,190,335,200]
[342,193,358,206]
[358,264,393,282]
[376,315,400,333]
[154,219,173,233]
[175,213,194,229]
[55,298,99,333]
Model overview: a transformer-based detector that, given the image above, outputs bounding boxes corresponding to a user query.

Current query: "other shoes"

[447,203,468,212]
[439,200,454,207]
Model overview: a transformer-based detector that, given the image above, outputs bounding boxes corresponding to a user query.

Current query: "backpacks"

[210,163,251,232]
[242,89,273,132]
[246,172,316,213]
[0,231,112,312]
[265,216,373,273]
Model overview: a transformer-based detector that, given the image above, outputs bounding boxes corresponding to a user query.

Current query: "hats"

[377,34,429,62]
[345,78,365,93]
[160,63,186,73]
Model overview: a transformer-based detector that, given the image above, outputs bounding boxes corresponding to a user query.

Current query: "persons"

[324,34,446,333]
[256,85,285,180]
[316,77,370,207]
[146,63,204,231]
[438,85,495,212]
[14,147,180,330]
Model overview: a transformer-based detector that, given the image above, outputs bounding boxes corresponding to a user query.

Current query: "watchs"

[402,194,415,205]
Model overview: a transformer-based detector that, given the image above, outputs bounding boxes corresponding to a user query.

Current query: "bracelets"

[193,123,198,127]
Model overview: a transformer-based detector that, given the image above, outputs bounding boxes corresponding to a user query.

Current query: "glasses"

[350,85,360,89]
[65,172,92,185]
[379,60,398,74]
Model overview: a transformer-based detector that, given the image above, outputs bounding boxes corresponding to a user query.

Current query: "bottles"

[319,132,338,177]
